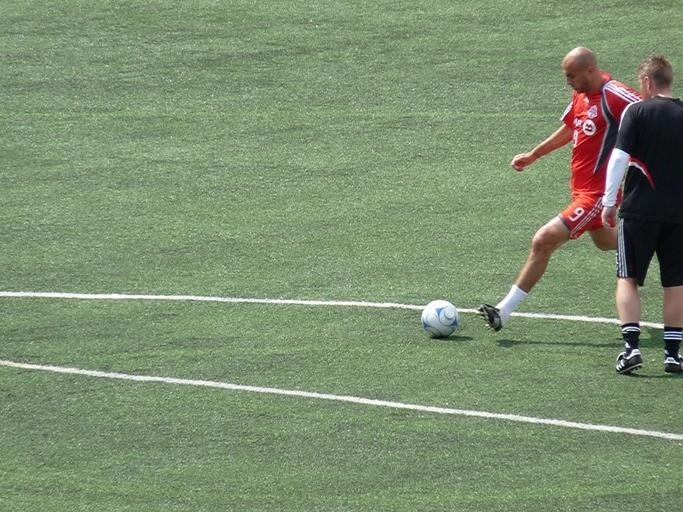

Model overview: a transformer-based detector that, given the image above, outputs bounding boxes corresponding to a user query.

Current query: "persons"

[601,54,683,375]
[478,47,645,331]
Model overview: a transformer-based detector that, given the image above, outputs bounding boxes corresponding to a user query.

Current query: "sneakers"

[664,352,683,373]
[615,348,644,374]
[478,303,504,333]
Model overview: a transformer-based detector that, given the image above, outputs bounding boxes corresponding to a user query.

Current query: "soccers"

[421,300,460,336]
